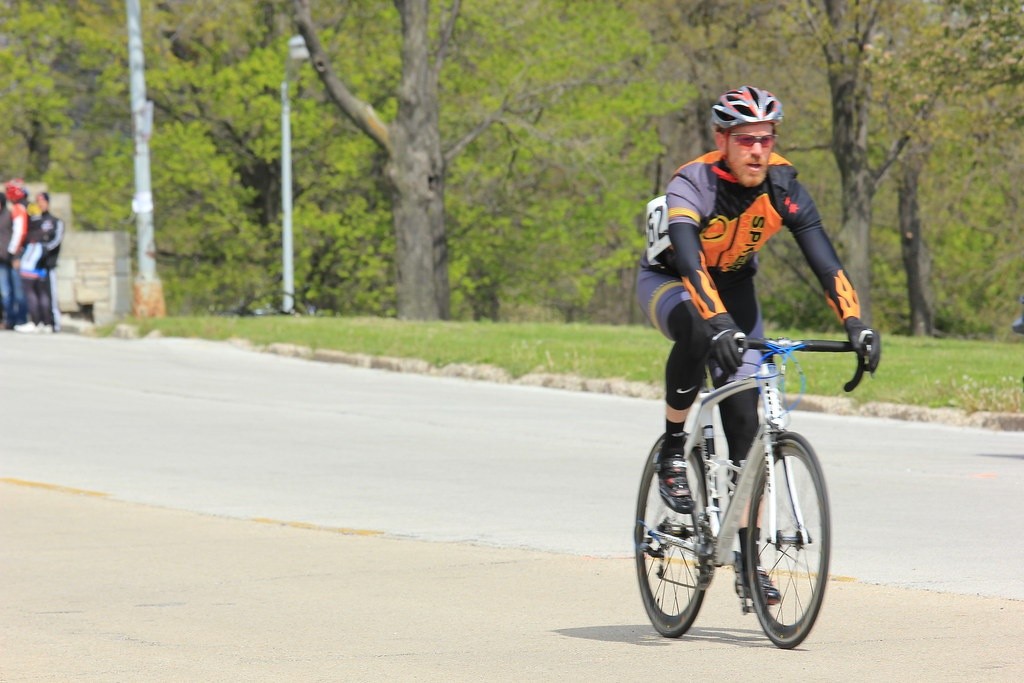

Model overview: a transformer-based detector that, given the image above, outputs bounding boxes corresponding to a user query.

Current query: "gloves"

[845,316,880,373]
[703,312,746,375]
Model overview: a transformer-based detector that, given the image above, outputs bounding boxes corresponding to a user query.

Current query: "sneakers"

[657,448,693,514]
[735,565,781,605]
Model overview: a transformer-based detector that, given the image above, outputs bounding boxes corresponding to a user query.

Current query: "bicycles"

[633,332,877,649]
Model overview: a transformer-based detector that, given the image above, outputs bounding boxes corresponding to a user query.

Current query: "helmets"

[4,179,27,202]
[711,85,784,129]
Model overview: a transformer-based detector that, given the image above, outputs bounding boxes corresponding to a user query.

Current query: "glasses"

[725,134,778,147]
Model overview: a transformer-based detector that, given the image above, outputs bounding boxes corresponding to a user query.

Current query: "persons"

[0,180,31,331]
[14,190,64,331]
[636,84,884,605]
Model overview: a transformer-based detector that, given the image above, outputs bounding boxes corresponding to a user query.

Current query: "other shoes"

[13,321,45,333]
[45,324,53,333]
